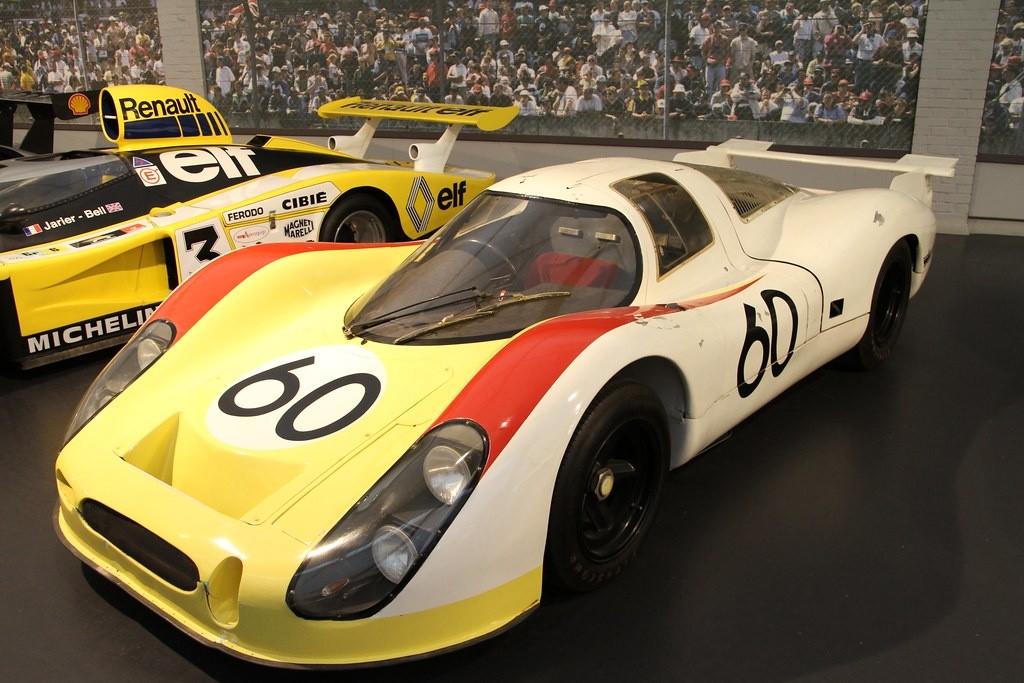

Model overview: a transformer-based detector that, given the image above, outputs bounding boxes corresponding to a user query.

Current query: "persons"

[0,0,164,93]
[982,0,1024,135]
[194,0,927,126]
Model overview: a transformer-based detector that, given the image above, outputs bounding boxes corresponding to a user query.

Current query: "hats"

[603,14,611,22]
[907,29,918,38]
[839,80,848,86]
[814,65,824,73]
[473,85,482,93]
[738,71,751,79]
[396,86,404,95]
[297,65,308,74]
[520,90,529,96]
[804,77,814,85]
[314,86,326,93]
[672,84,685,93]
[670,56,684,64]
[886,30,898,38]
[721,79,730,88]
[500,39,509,45]
[858,91,873,101]
[109,16,117,21]
[281,65,289,71]
[418,18,427,23]
[539,5,549,11]
[304,11,311,16]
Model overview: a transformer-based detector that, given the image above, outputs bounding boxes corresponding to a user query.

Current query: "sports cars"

[51,136,958,671]
[0,83,524,374]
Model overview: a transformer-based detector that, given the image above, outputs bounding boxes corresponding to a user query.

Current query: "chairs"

[531,256,619,292]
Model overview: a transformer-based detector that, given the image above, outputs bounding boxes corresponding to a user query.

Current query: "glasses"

[589,60,595,62]
[607,92,614,95]
[823,96,832,102]
